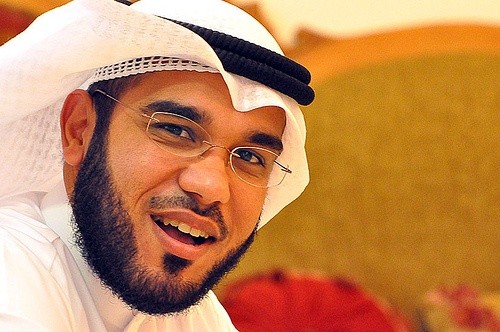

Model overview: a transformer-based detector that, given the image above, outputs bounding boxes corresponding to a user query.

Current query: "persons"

[0,0,315,332]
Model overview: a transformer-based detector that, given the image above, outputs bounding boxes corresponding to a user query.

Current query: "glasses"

[93,90,292,189]
